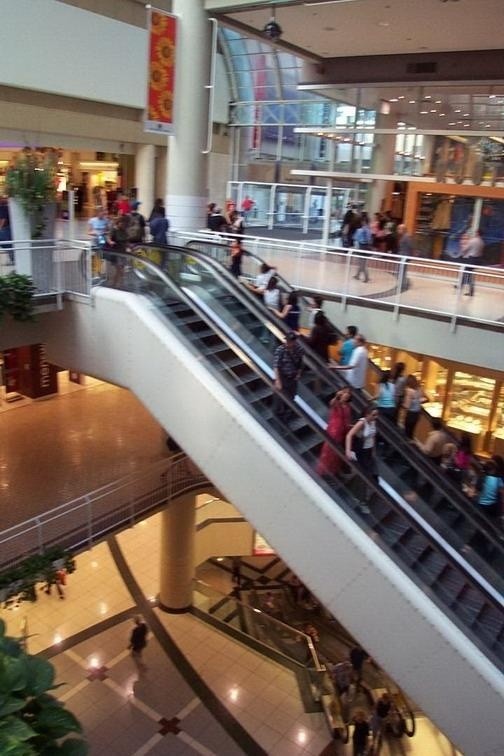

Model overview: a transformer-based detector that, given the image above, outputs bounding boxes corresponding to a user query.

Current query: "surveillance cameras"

[264,23,283,39]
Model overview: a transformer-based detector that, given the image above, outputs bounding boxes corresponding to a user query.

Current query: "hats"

[284,333,299,340]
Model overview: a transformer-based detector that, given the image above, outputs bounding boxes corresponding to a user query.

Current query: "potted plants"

[0,145,64,292]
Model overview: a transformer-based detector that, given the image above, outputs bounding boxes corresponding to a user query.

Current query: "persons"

[332,643,400,756]
[273,332,305,414]
[208,200,243,240]
[240,264,281,309]
[327,324,422,439]
[353,217,373,282]
[231,240,241,277]
[405,417,504,518]
[161,436,196,479]
[241,196,255,222]
[249,574,339,636]
[315,385,381,517]
[454,229,485,296]
[87,190,169,288]
[45,567,64,598]
[0,194,15,265]
[232,556,242,585]
[127,615,148,670]
[341,200,397,260]
[292,293,337,361]
[267,294,301,329]
[296,623,319,663]
[436,139,463,182]
[396,224,414,289]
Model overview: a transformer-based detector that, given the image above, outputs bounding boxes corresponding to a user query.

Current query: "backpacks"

[127,213,142,242]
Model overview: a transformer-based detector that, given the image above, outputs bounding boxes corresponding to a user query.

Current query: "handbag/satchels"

[353,437,363,450]
